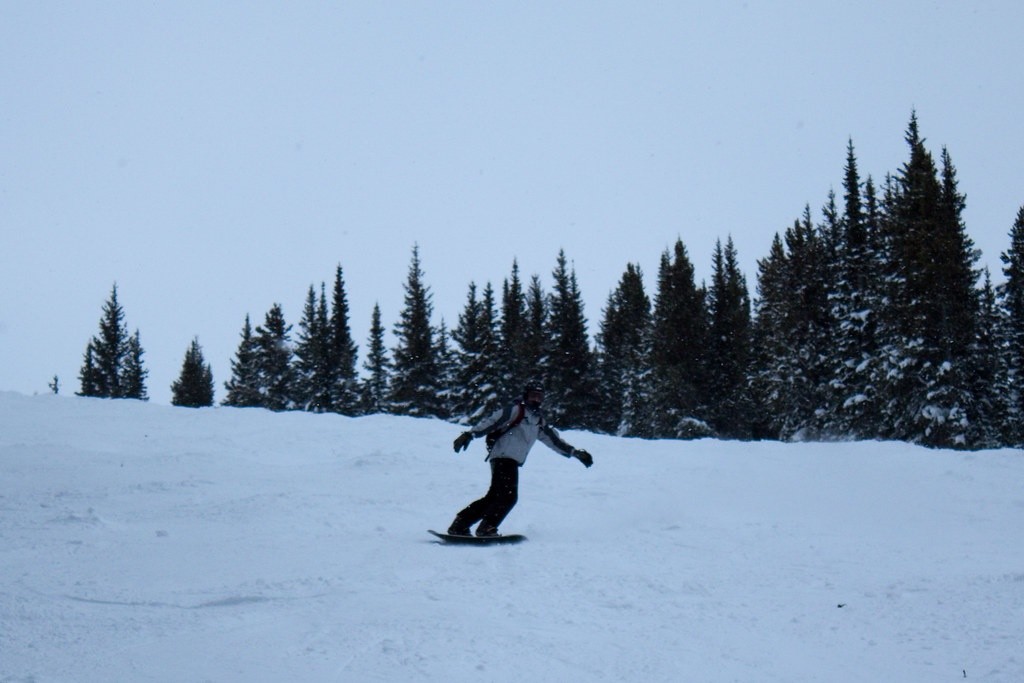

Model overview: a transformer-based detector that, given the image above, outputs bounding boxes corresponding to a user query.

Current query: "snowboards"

[426,529,524,543]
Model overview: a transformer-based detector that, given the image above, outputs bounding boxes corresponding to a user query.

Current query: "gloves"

[574,449,594,468]
[453,432,473,453]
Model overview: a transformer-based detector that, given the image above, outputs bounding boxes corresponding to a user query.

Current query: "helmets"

[523,380,546,409]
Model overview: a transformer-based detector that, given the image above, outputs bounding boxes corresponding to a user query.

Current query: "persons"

[447,384,593,538]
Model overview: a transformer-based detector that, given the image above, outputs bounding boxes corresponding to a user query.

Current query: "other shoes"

[447,515,474,536]
[475,525,500,536]
